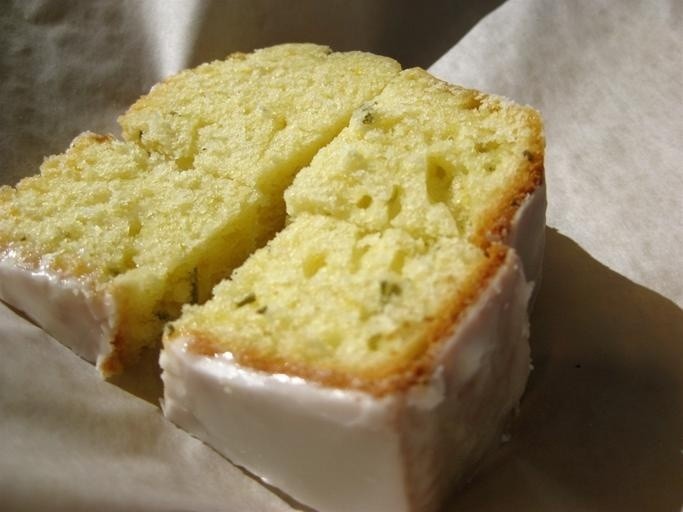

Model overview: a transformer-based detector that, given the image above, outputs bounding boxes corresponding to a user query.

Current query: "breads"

[0,42,547,512]
